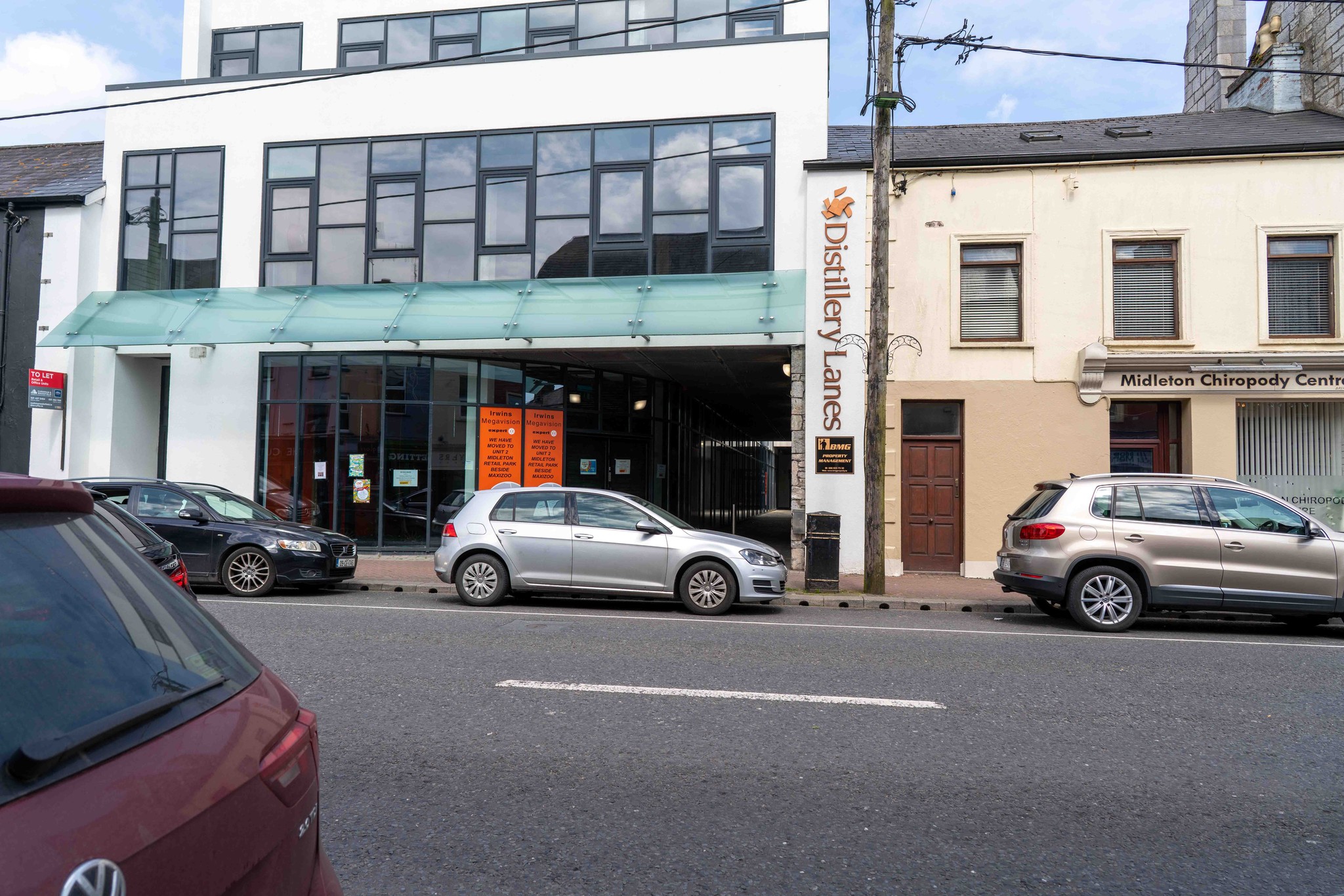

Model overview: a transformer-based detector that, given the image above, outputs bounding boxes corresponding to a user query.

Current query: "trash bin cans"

[802,511,842,592]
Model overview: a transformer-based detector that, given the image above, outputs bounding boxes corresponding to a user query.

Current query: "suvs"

[991,471,1344,633]
[0,470,346,896]
[432,481,790,616]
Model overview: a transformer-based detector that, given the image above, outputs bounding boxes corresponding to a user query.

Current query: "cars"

[257,469,476,548]
[58,475,359,598]
